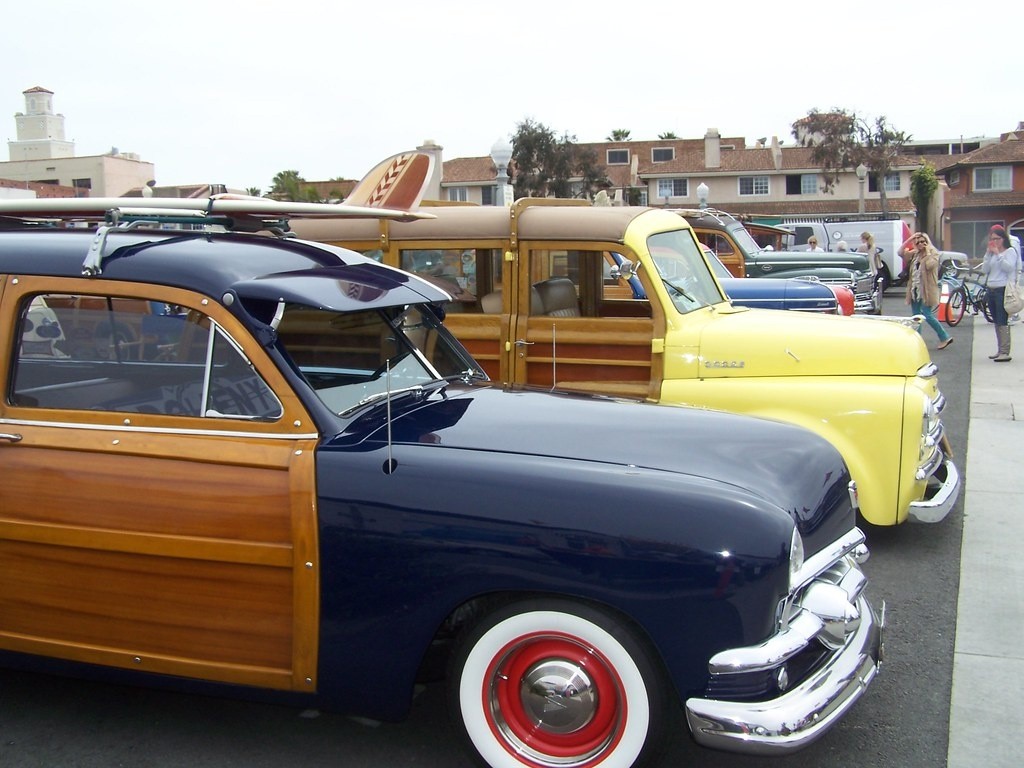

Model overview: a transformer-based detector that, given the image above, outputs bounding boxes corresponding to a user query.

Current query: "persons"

[981,225,1022,363]
[897,232,954,350]
[805,233,878,282]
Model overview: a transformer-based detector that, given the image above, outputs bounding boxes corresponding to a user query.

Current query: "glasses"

[810,242,816,244]
[915,240,926,245]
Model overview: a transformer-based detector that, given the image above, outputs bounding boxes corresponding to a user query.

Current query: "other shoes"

[936,338,953,350]
[994,353,1012,362]
[989,353,1000,359]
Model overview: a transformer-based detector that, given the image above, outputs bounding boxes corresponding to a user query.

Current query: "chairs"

[481,278,580,318]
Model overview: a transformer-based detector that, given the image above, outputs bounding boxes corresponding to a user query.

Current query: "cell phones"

[988,241,995,248]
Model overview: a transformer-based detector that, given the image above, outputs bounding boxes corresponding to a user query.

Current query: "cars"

[0,192,892,768]
[532,203,886,317]
[273,139,962,541]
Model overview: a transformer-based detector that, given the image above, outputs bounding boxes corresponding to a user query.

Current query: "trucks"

[772,219,915,291]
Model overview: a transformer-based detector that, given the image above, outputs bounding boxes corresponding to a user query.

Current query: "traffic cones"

[936,281,957,322]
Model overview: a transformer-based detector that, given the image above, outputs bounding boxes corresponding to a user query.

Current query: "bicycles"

[930,255,1014,324]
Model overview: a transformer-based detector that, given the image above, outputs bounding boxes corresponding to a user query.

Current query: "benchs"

[12,374,280,419]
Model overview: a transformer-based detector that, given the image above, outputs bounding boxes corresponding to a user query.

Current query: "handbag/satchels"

[1002,281,1024,315]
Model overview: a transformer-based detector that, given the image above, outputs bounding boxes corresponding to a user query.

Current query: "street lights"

[854,162,874,214]
